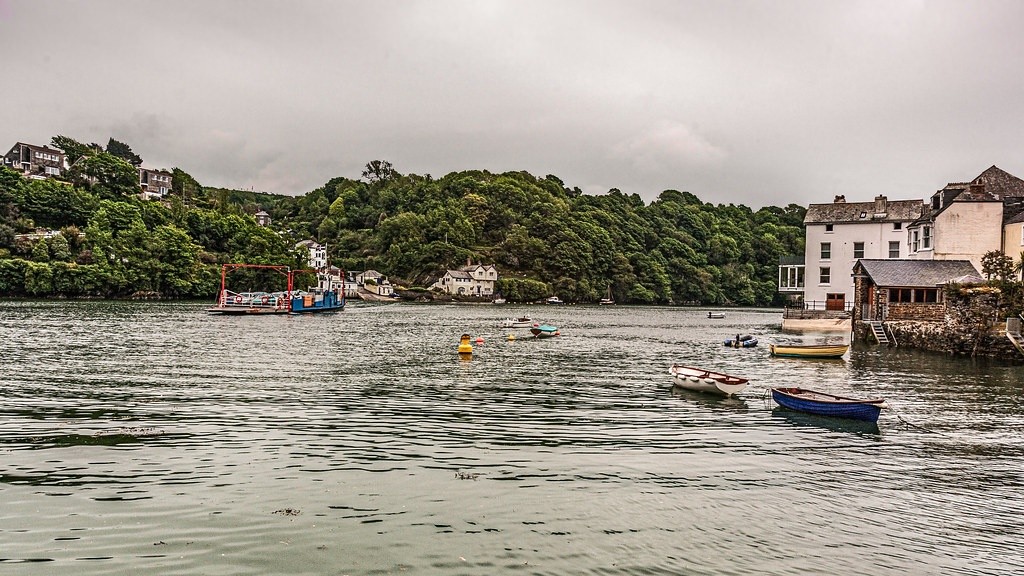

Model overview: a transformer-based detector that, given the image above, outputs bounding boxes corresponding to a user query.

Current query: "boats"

[770,344,850,359]
[496,316,532,327]
[546,296,564,305]
[706,313,726,318]
[772,387,886,422]
[291,269,347,313]
[459,333,472,354]
[724,336,758,347]
[669,363,750,396]
[530,324,560,337]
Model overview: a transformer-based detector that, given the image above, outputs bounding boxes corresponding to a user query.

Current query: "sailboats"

[598,285,616,305]
[203,264,293,315]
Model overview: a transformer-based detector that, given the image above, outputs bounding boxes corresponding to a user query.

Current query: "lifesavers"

[236,295,242,302]
[262,296,268,304]
